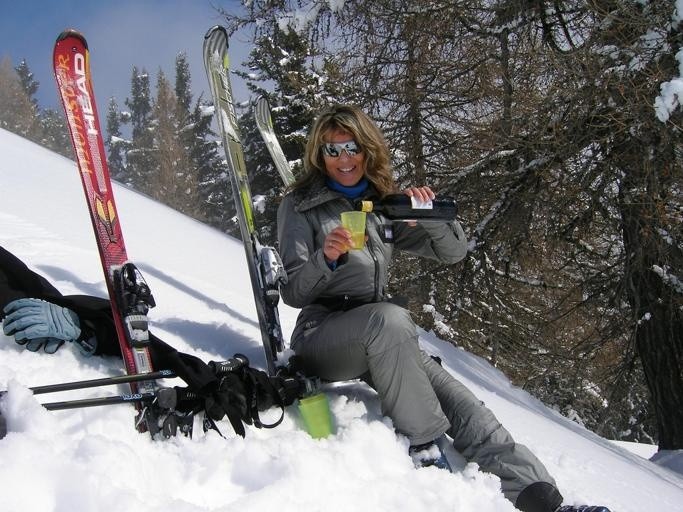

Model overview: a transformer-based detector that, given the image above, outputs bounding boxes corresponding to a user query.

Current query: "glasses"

[322,140,363,157]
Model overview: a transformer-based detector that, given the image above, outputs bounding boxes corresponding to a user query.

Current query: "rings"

[328,240,333,247]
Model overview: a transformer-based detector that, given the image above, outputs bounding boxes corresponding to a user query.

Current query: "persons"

[276,104,563,512]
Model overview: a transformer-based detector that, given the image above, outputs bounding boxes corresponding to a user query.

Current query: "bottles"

[355,195,459,221]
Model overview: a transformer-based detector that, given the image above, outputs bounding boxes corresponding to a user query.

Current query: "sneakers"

[557,505,610,512]
[409,441,451,471]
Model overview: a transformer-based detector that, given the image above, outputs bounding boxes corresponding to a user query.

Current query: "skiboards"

[204,25,320,406]
[54,27,157,435]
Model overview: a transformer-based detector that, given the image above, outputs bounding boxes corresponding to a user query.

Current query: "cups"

[297,391,332,440]
[341,210,367,251]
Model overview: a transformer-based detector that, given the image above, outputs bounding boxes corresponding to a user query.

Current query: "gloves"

[3,298,81,353]
